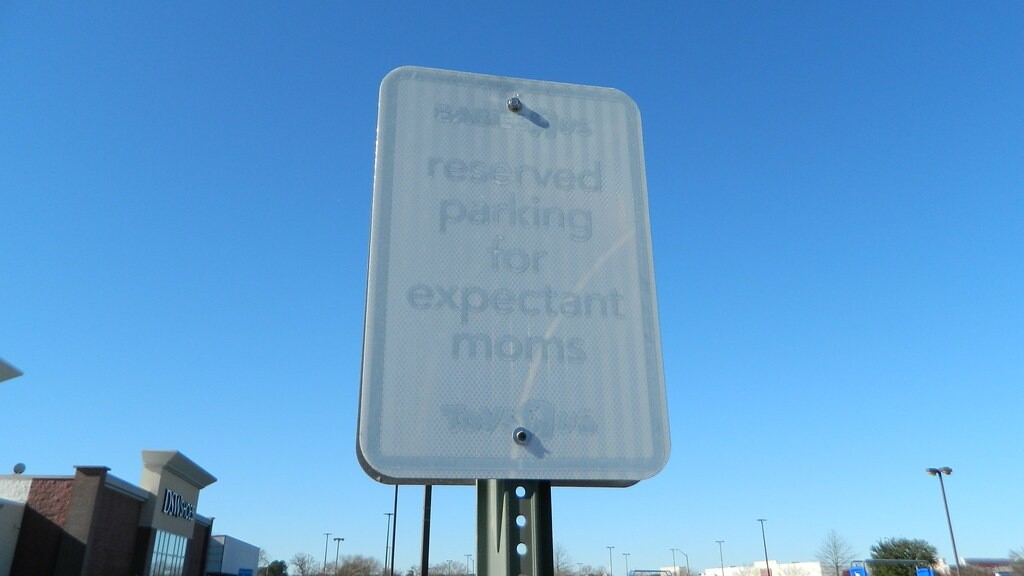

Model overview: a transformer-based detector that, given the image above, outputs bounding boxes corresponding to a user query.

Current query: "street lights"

[464,554,473,576]
[926,466,960,576]
[675,548,690,576]
[576,562,584,576]
[607,546,615,576]
[383,512,395,575]
[757,519,770,576]
[669,548,676,576]
[323,532,332,576]
[622,552,631,576]
[333,538,345,575]
[716,540,724,576]
